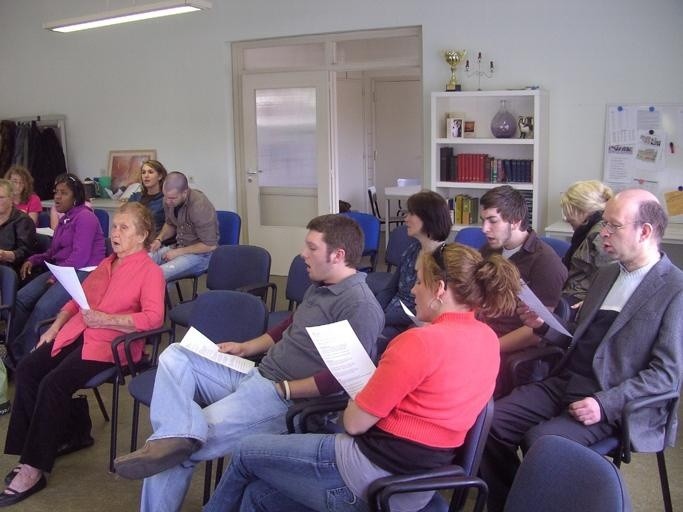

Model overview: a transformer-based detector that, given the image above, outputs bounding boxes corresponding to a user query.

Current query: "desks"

[384,184,422,250]
[40,197,128,239]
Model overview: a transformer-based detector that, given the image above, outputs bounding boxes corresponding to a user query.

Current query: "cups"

[99,176,112,198]
[82,182,94,199]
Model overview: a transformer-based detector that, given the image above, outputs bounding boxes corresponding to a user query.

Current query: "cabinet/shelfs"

[430,88,549,243]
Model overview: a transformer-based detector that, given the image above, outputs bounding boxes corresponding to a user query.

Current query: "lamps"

[42,0,211,35]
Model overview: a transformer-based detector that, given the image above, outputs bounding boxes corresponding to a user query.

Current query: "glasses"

[434,241,447,294]
[54,175,76,185]
[597,220,636,233]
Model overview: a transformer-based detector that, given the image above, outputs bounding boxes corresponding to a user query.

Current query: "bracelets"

[282,379,291,400]
[152,238,161,241]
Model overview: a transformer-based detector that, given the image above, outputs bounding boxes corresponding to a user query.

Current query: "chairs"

[368,185,407,263]
[396,178,423,228]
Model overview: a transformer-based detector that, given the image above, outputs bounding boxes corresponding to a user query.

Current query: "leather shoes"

[0,476,47,507]
[5,467,22,483]
[114,437,194,480]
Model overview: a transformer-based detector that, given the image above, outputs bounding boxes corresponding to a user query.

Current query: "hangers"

[15,116,59,129]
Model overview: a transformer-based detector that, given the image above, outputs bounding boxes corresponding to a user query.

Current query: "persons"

[0,202,165,504]
[201,244,521,512]
[115,214,386,511]
[478,183,569,400]
[487,188,683,511]
[121,161,166,233]
[147,171,220,281]
[377,191,451,340]
[0,166,107,361]
[561,179,614,323]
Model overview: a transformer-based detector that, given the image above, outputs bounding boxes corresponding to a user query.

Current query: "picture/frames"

[447,118,464,139]
[105,146,158,203]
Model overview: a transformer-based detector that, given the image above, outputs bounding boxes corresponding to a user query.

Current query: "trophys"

[440,48,467,91]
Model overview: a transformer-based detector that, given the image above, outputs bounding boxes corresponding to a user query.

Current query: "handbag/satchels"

[54,396,94,455]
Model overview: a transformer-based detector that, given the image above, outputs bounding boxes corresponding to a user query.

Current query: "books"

[438,147,533,229]
